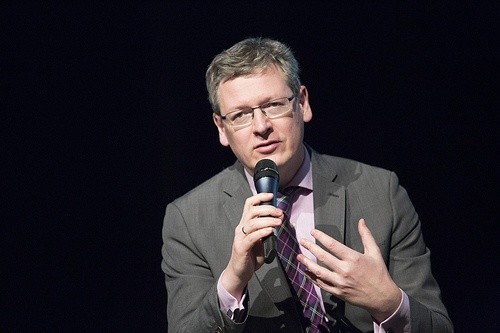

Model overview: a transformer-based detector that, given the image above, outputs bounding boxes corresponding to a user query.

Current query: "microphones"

[254,159,280,264]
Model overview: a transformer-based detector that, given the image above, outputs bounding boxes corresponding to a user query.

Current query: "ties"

[273,186,330,333]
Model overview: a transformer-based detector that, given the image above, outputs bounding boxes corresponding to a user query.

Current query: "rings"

[242,226,248,236]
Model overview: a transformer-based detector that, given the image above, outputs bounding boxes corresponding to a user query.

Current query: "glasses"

[220,91,297,128]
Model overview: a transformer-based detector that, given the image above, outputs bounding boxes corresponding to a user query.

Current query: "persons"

[161,37,455,333]
